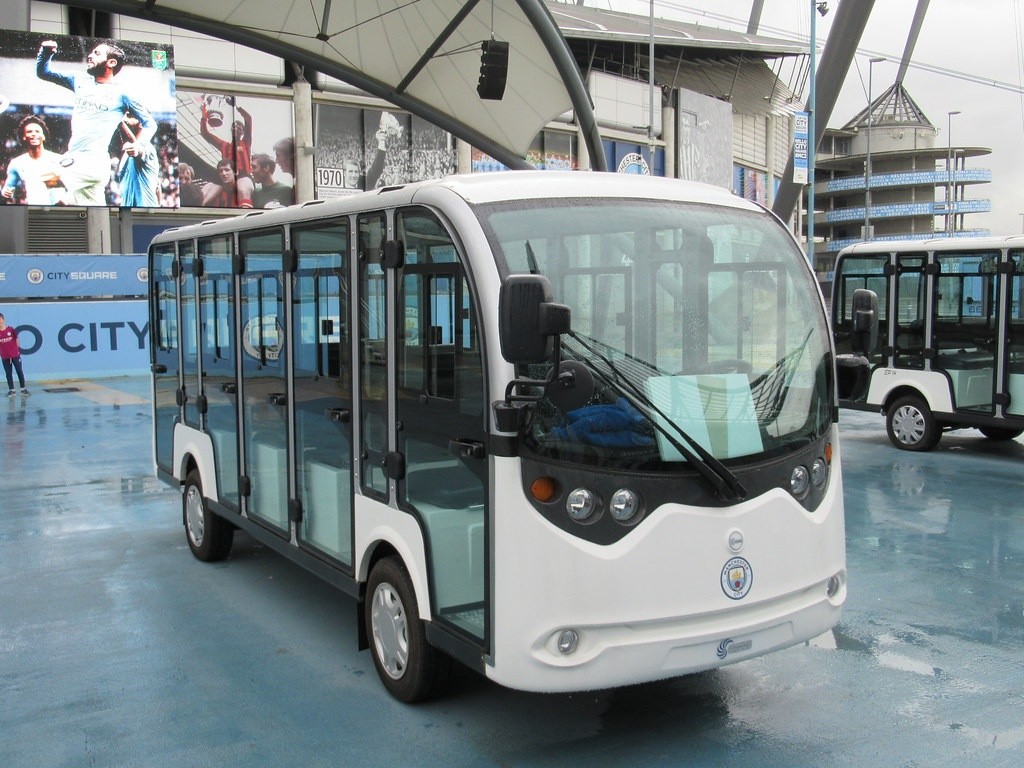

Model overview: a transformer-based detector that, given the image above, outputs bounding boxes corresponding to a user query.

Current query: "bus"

[143,165,882,705]
[827,234,1024,452]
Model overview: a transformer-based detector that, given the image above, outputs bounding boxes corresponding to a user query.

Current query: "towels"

[551,397,656,447]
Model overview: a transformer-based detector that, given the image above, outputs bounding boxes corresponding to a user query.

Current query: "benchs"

[208,420,486,617]
[834,312,1022,370]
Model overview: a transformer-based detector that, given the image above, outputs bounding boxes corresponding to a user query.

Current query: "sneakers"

[6,391,16,397]
[20,389,31,396]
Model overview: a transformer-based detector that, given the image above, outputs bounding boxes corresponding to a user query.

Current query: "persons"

[0,314,29,398]
[0,44,296,209]
[316,110,459,197]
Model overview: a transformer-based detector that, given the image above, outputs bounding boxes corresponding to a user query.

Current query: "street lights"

[947,110,961,237]
[862,57,887,242]
